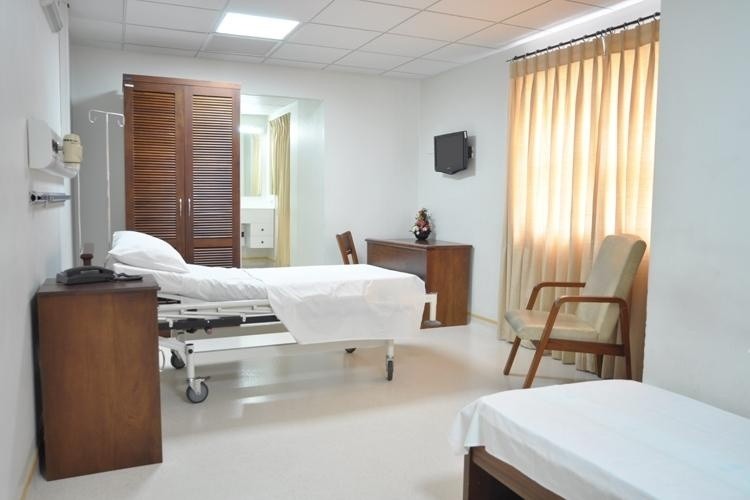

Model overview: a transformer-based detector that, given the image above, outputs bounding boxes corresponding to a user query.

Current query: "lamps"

[53,134,83,178]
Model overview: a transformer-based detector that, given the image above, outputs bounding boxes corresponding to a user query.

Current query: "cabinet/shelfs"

[122,72,240,270]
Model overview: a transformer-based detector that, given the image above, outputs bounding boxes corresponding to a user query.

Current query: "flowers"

[409,208,432,234]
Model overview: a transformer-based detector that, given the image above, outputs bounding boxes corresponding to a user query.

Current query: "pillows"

[109,228,189,274]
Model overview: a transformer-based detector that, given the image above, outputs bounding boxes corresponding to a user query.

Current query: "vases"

[415,230,430,240]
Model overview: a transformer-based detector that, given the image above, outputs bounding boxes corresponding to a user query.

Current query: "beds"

[113,259,427,403]
[457,378,749,500]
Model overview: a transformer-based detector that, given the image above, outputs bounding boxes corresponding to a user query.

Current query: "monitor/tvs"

[433,131,468,175]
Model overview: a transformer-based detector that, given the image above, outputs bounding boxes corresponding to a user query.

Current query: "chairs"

[501,232,647,390]
[336,230,359,265]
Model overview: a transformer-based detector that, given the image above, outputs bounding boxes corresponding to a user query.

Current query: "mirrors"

[240,113,269,198]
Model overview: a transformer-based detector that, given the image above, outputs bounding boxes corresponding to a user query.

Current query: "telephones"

[56,266,115,285]
